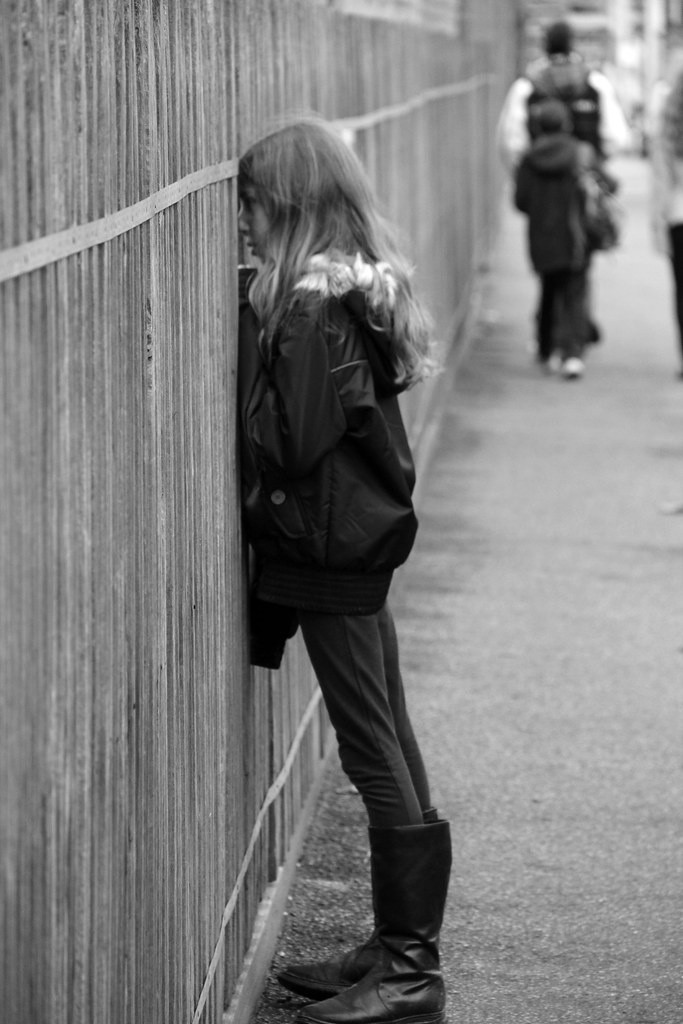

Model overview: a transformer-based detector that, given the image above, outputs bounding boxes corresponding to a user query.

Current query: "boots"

[279,809,452,1019]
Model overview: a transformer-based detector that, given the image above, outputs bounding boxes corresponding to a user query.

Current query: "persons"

[657,62,683,376]
[496,21,634,344]
[237,124,454,1024]
[511,97,618,378]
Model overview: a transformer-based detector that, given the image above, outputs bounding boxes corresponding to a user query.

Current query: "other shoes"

[545,348,585,376]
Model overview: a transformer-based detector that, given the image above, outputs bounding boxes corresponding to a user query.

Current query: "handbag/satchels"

[583,165,616,248]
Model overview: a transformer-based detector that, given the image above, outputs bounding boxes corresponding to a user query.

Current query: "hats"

[546,22,572,55]
